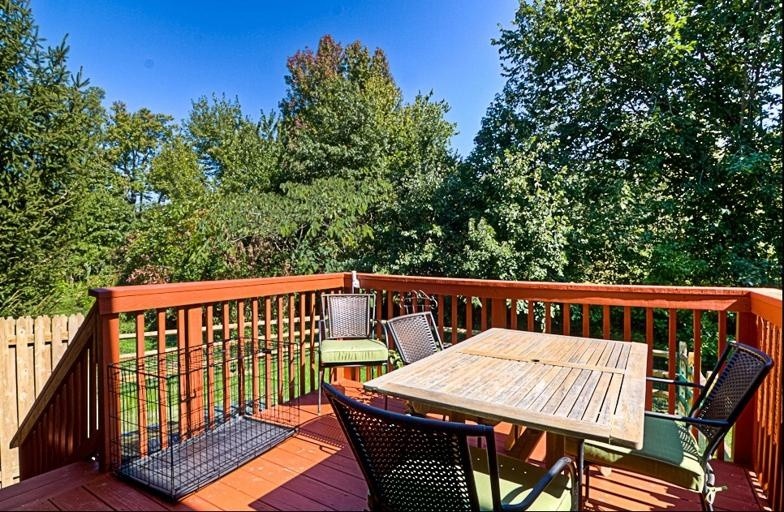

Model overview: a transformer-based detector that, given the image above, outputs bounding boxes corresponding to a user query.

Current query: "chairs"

[323,384,579,512]
[386,311,502,447]
[318,294,390,414]
[578,342,774,512]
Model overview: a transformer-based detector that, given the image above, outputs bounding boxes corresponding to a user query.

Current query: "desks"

[363,327,648,472]
